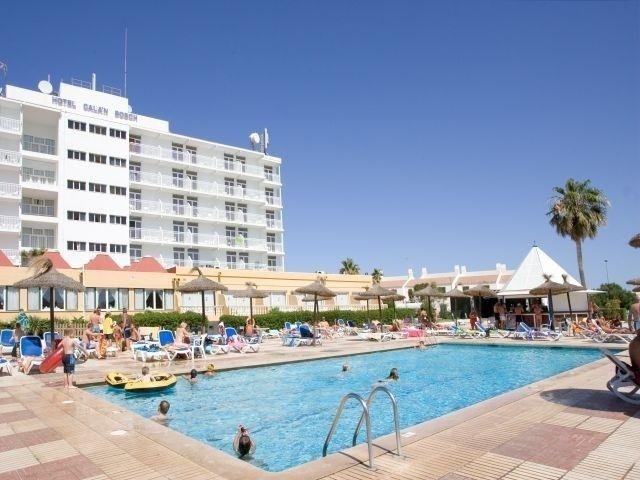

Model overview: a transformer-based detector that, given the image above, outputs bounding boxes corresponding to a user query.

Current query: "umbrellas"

[624,277,640,293]
[555,275,585,323]
[530,276,570,332]
[413,285,441,330]
[233,283,269,326]
[629,233,640,248]
[176,268,228,334]
[442,286,468,330]
[354,278,406,333]
[464,284,497,324]
[14,258,86,345]
[295,281,337,345]
[301,294,332,323]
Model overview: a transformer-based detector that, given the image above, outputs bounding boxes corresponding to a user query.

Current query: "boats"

[103,371,132,386]
[124,372,178,391]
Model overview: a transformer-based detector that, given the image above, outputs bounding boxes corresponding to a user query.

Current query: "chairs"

[601,347,640,406]
[282,320,426,348]
[428,319,511,339]
[0,327,260,377]
[520,317,634,345]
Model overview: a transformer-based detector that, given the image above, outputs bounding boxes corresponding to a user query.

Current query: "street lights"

[603,258,612,301]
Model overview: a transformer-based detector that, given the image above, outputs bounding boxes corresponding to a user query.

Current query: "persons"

[233,425,256,461]
[469,307,478,330]
[150,401,173,423]
[490,301,544,331]
[586,295,640,386]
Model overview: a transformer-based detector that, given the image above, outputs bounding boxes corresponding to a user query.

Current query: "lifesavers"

[39,346,65,374]
